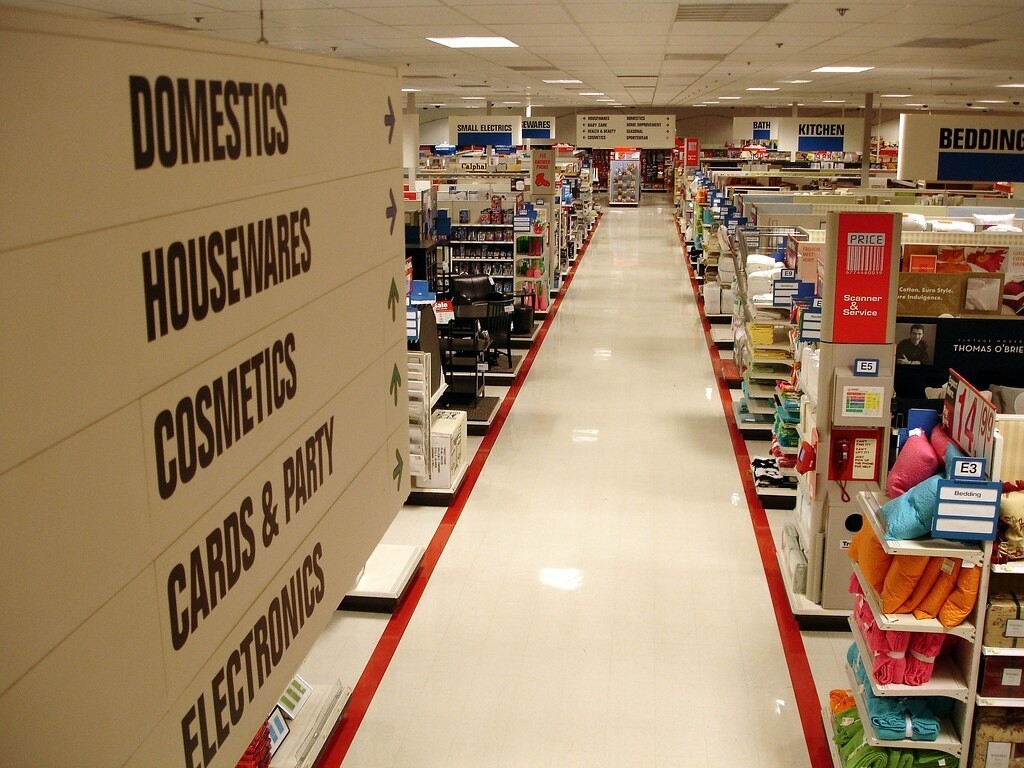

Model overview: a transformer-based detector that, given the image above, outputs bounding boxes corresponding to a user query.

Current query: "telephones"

[830,427,881,480]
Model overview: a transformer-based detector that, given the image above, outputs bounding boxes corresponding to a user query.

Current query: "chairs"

[482,298,513,371]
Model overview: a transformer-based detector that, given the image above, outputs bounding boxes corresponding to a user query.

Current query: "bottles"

[516,236,543,256]
[516,278,550,311]
[517,259,544,277]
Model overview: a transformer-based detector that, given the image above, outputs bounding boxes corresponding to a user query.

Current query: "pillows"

[902,213,927,231]
[928,219,975,232]
[973,213,1015,227]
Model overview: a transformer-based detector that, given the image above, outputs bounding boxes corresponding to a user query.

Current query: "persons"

[896,324,929,365]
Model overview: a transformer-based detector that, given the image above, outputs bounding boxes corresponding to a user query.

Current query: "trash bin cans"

[823,505,866,611]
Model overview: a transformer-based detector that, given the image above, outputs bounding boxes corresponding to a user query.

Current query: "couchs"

[450,274,507,329]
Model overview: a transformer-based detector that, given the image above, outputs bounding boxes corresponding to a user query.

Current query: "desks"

[504,291,536,328]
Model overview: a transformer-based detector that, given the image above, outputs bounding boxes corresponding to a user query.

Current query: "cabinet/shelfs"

[404,183,565,299]
[825,414,1024,768]
[674,138,1024,389]
[570,187,584,254]
[404,191,556,321]
[437,318,493,409]
[405,240,441,292]
[743,225,811,511]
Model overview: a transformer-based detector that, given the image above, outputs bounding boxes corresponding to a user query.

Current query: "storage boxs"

[988,588,1024,600]
[970,717,1024,768]
[977,656,1024,698]
[415,408,468,489]
[983,599,1024,648]
[567,239,577,259]
[450,209,514,293]
[560,246,569,272]
